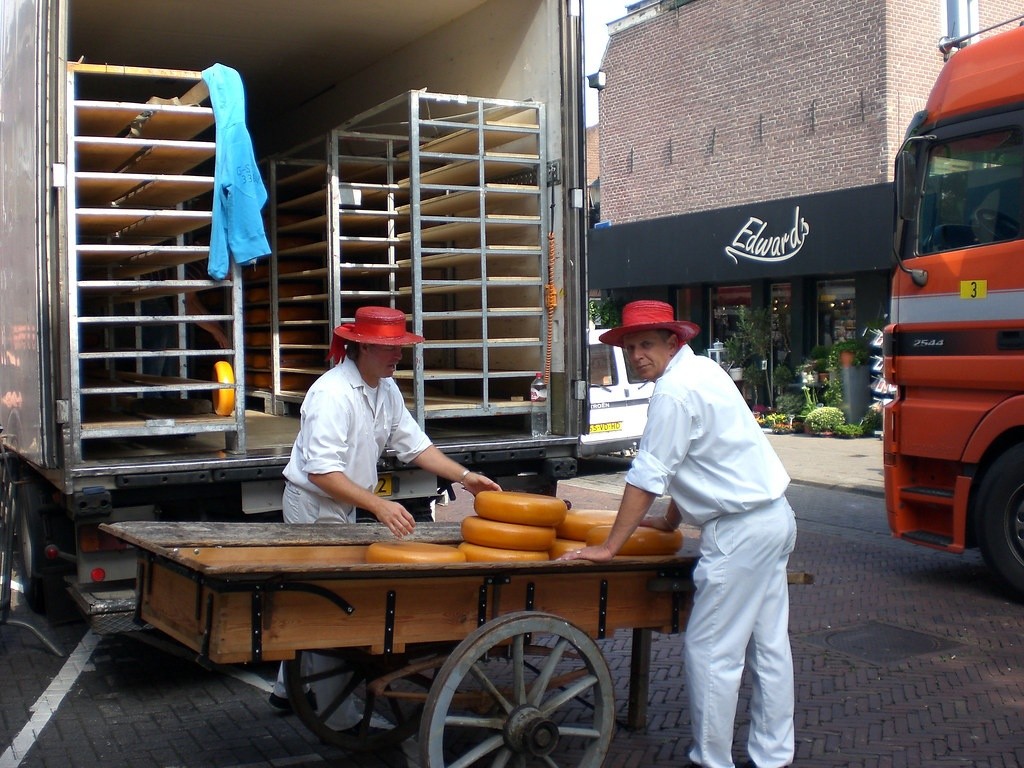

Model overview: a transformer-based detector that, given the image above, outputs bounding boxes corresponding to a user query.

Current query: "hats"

[325,306,425,363]
[599,299,703,347]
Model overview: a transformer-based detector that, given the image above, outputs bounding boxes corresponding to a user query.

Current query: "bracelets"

[459,470,470,483]
[664,516,674,530]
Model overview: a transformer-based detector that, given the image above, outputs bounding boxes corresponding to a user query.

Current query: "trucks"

[0,1,590,686]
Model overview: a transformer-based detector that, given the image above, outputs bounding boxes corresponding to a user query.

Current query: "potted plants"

[588,296,619,328]
[724,306,885,438]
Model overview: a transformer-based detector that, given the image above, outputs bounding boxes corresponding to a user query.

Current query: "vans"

[578,329,656,471]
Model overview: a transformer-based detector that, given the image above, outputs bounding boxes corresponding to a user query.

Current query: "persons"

[267,306,501,746]
[120,237,230,400]
[558,300,799,768]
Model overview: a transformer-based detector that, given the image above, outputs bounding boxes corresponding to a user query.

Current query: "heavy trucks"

[881,15,1024,593]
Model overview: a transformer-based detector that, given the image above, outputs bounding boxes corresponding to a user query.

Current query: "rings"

[577,550,581,554]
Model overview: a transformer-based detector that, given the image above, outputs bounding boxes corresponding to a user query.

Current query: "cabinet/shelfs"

[65,62,552,463]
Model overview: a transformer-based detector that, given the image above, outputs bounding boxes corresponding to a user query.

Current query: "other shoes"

[269,688,318,711]
[336,718,387,737]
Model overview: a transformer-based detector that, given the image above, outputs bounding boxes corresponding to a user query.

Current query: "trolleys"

[95,518,812,762]
